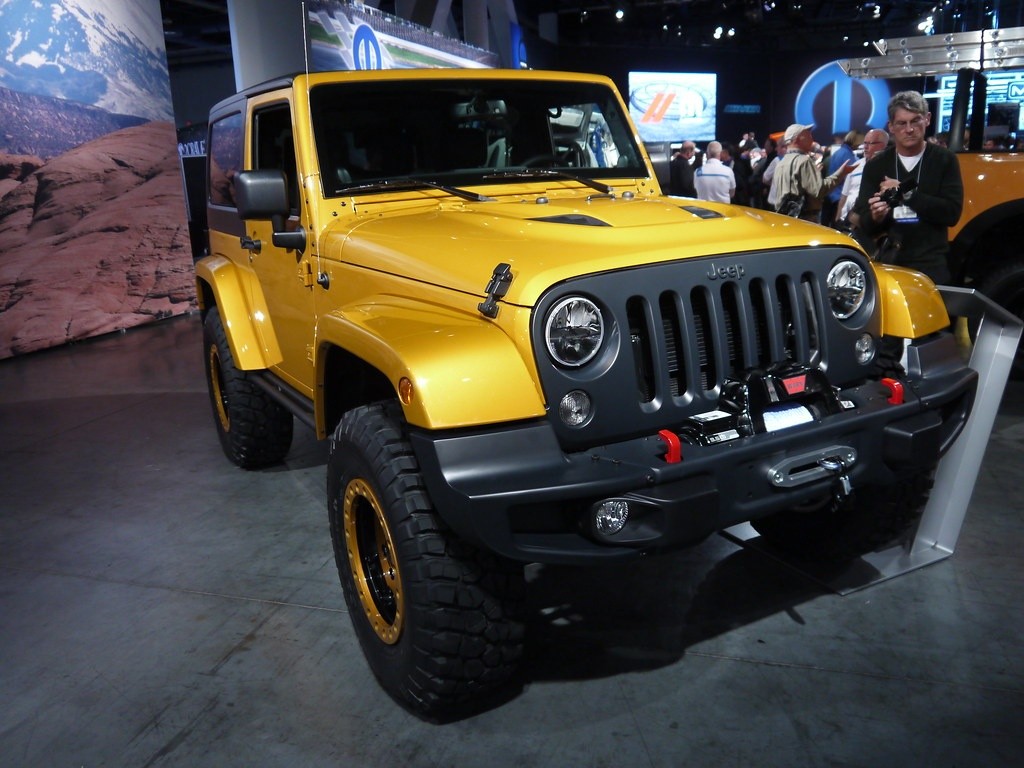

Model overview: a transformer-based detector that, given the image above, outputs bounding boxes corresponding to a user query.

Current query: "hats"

[784,124,814,142]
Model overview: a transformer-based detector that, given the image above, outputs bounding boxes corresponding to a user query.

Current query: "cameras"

[881,176,916,209]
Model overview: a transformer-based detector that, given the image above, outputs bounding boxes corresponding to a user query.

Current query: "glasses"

[864,141,881,147]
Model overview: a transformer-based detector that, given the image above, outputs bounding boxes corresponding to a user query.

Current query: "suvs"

[193,61,979,727]
[938,68,1024,383]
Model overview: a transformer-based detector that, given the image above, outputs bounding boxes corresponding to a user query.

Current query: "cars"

[644,141,701,186]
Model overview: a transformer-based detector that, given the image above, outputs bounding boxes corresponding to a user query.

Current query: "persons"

[671,123,890,233]
[928,128,1024,152]
[850,91,966,271]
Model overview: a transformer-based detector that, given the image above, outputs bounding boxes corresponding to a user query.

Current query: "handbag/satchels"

[776,154,817,219]
